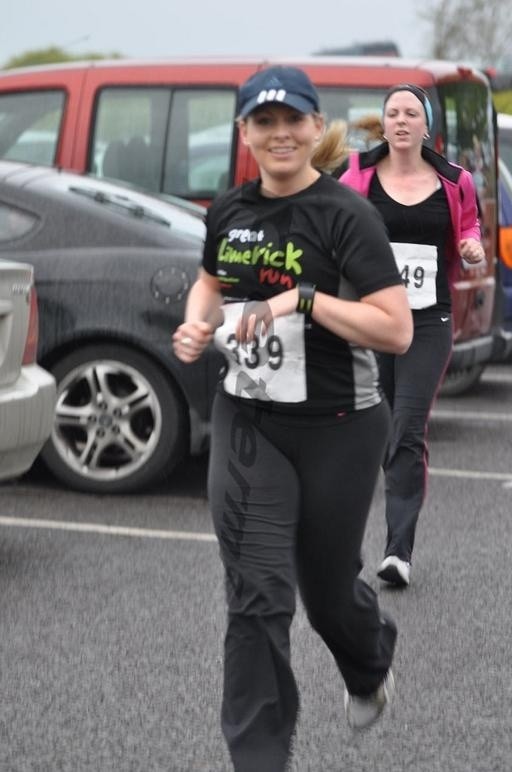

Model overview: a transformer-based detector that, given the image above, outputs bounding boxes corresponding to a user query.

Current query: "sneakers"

[375,552,411,586]
[343,610,398,733]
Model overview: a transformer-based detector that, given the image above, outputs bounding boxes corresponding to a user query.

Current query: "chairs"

[102,133,149,184]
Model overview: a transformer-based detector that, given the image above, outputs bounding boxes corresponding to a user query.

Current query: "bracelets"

[297,281,316,316]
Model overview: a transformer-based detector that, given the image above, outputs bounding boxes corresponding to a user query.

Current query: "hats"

[231,63,321,124]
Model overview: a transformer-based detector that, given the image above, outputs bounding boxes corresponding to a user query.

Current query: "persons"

[171,66,418,772]
[327,84,486,587]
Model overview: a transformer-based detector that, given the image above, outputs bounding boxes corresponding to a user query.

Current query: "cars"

[1,259,61,480]
[3,162,229,495]
[2,56,512,399]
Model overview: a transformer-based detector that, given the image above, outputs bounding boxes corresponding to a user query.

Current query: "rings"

[181,336,191,347]
